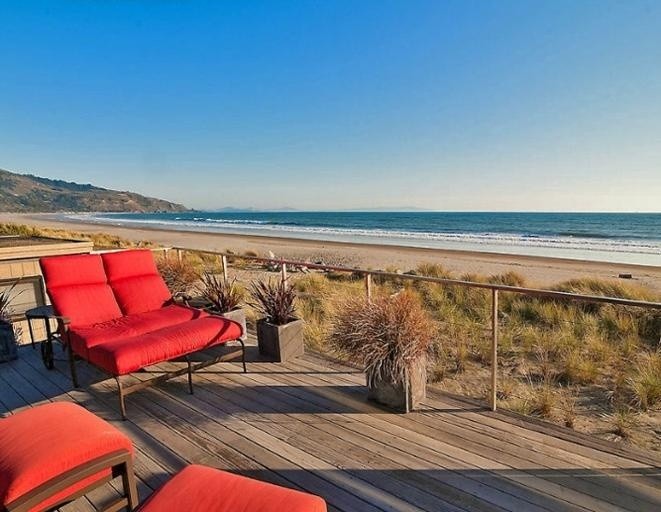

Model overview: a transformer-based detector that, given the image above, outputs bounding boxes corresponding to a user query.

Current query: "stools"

[25,304,60,349]
[0,400,139,512]
[133,461,329,512]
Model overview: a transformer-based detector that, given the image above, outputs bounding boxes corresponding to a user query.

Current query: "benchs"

[38,249,247,421]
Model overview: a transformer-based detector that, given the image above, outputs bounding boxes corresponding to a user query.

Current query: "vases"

[1,318,19,363]
[203,306,247,348]
[365,354,429,412]
[256,314,304,362]
